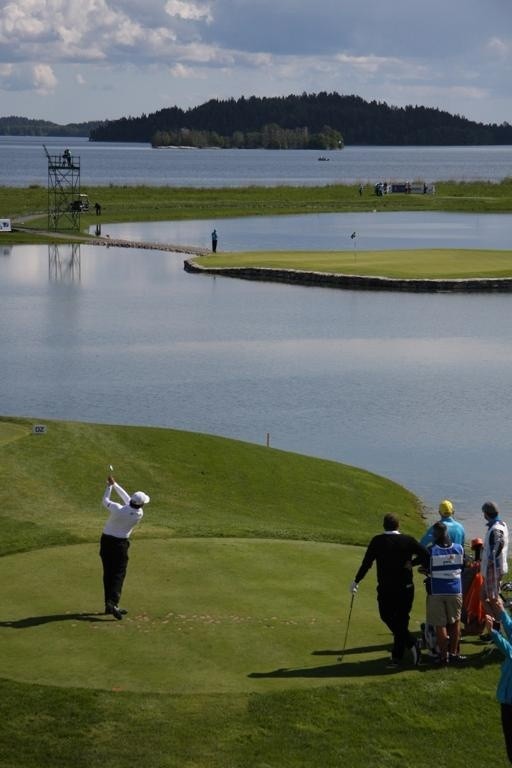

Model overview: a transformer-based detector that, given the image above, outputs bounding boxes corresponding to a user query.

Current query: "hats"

[131,491,150,505]
[438,499,452,517]
[470,538,483,550]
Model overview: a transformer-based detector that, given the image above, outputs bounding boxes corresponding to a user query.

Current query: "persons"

[67,149,71,167]
[485,593,512,765]
[211,229,217,254]
[351,501,509,671]
[99,475,150,621]
[95,203,101,216]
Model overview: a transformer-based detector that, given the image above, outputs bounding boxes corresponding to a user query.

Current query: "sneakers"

[105,600,127,620]
[410,638,422,665]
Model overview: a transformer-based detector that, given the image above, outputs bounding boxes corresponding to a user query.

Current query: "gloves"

[350,581,358,593]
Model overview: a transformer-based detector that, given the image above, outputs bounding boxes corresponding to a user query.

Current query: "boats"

[318,158,330,161]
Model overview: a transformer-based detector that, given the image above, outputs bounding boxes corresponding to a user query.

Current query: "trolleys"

[412,566,444,665]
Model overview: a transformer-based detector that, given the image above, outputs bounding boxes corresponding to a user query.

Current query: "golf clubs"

[337,594,353,662]
